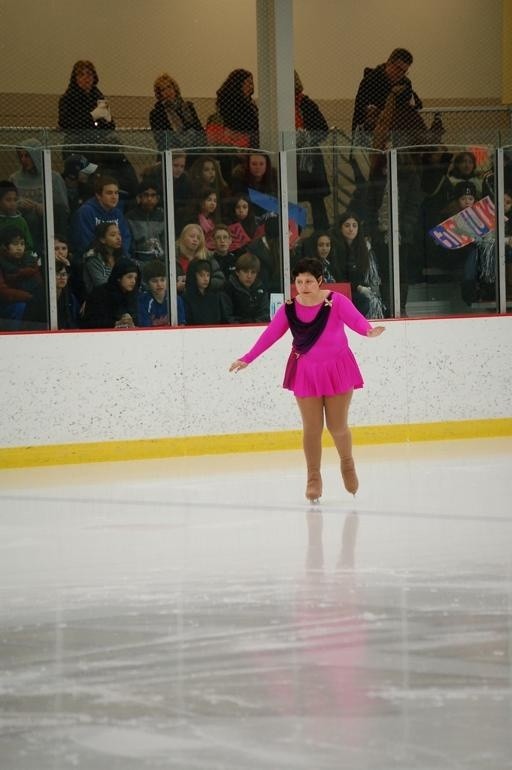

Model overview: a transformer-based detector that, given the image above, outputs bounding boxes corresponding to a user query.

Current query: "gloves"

[90,104,112,122]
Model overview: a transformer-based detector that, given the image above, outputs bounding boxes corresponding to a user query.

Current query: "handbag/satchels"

[206,112,251,151]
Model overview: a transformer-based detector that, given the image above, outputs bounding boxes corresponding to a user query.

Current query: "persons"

[228,257,386,500]
[0,47,512,330]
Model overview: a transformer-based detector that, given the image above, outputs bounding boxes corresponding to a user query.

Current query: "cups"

[97,99,110,107]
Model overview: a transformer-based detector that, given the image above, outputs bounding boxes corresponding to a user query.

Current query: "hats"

[456,182,476,198]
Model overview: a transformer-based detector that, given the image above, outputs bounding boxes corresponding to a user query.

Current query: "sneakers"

[305,469,322,499]
[341,466,359,492]
[306,510,323,532]
[342,513,359,536]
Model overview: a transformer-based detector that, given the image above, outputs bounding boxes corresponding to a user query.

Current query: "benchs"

[420,265,511,311]
